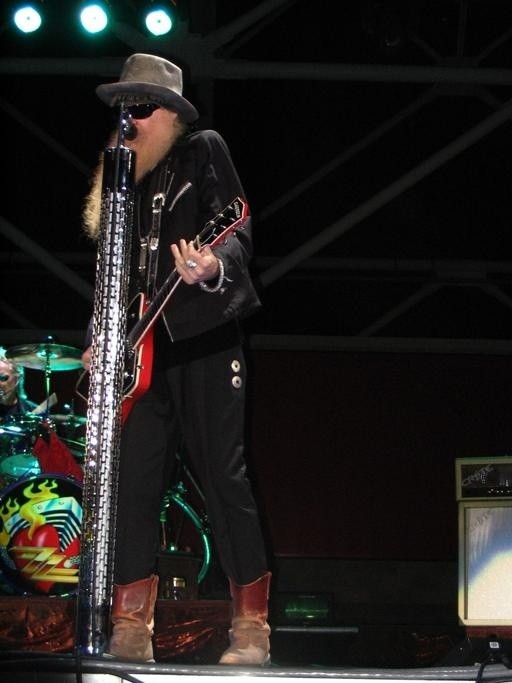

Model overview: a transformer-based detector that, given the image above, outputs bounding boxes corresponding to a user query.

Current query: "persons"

[78,53,278,667]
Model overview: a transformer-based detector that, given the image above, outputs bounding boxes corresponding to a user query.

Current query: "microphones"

[122,102,138,140]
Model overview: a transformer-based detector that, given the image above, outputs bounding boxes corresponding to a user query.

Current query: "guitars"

[76,196,248,429]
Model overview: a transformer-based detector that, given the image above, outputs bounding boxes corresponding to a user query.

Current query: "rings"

[187,258,198,268]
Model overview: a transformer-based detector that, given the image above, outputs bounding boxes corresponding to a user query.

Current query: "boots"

[106,575,159,663]
[218,572,272,667]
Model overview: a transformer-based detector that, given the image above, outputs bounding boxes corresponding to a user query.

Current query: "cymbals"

[5,344,85,372]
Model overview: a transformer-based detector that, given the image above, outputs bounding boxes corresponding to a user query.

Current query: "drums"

[0,474,84,598]
[1,453,41,497]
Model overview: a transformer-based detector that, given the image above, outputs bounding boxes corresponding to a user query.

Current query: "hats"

[98,52,199,123]
